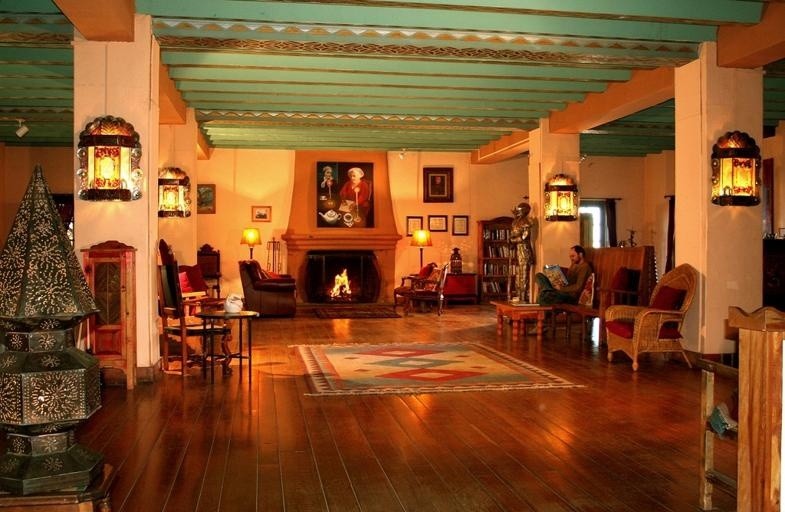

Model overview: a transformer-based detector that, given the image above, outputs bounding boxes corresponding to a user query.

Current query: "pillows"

[647,286,685,321]
[424,266,442,289]
[414,262,433,287]
[603,267,628,309]
[578,272,596,307]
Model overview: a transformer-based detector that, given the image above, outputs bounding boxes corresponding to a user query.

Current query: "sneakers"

[528,327,548,336]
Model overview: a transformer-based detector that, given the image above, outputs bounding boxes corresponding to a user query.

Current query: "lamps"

[240,228,262,259]
[78,115,142,202]
[160,166,190,220]
[410,229,433,268]
[543,171,579,221]
[713,131,761,207]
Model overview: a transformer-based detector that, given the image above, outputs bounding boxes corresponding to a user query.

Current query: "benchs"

[545,247,651,341]
[393,277,445,316]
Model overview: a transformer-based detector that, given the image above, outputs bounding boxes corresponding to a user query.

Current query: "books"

[483,229,518,292]
[543,262,569,289]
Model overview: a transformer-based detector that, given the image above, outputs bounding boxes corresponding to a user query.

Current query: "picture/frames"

[197,184,216,214]
[251,206,272,223]
[406,167,469,236]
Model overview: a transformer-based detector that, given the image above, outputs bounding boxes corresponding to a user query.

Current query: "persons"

[432,177,444,195]
[526,245,593,335]
[339,166,372,220]
[317,165,337,193]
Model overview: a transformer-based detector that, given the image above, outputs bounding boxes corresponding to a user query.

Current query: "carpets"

[314,305,402,319]
[288,341,587,397]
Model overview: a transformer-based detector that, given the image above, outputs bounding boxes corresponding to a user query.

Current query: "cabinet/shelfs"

[477,217,517,300]
[81,350,136,390]
[443,272,478,306]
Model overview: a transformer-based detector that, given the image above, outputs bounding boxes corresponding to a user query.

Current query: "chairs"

[157,240,232,384]
[603,263,699,373]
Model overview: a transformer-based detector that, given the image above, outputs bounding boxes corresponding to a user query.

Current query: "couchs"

[239,259,297,316]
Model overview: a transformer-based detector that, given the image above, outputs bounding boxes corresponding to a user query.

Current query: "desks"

[197,311,259,387]
